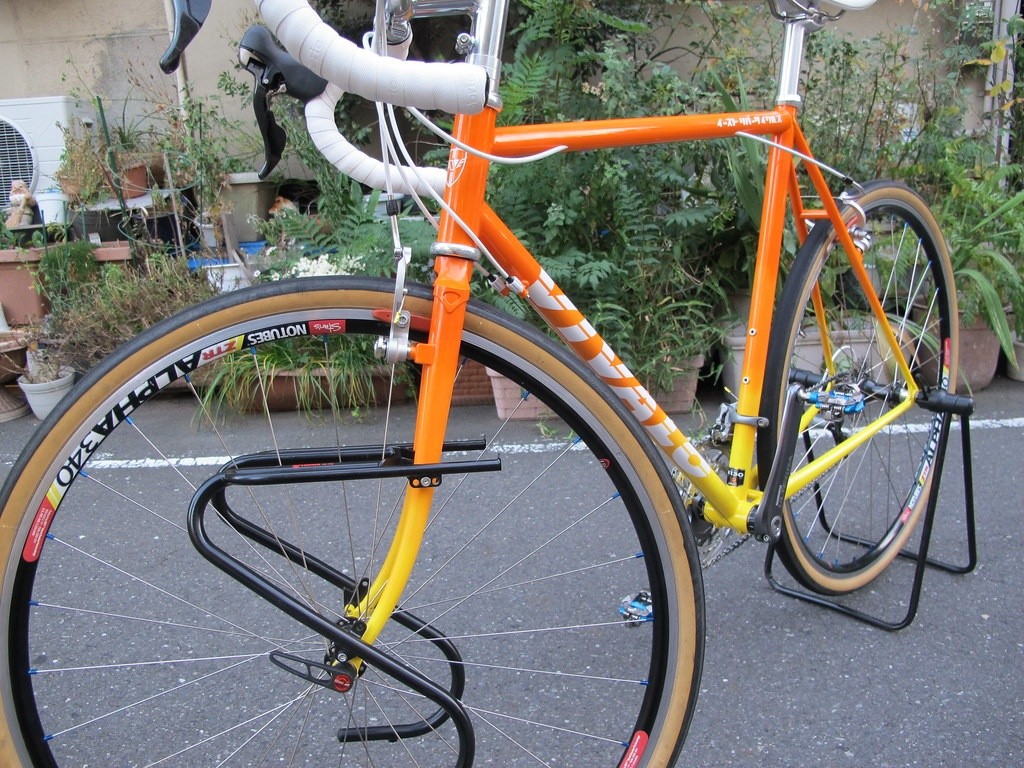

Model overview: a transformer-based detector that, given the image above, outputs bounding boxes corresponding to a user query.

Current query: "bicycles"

[0,0,979,768]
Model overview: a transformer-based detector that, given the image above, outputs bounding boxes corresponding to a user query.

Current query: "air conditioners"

[0,95,78,228]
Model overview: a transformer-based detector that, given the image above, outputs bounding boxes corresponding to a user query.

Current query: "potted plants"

[0,0,1024,422]
